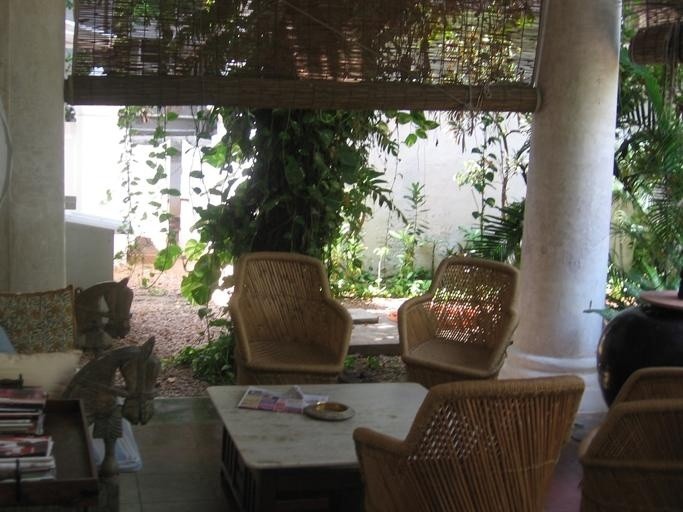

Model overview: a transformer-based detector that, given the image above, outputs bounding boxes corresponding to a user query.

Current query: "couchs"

[0,276,161,512]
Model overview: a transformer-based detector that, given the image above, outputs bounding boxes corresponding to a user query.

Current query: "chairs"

[398,255,521,390]
[576,367,683,512]
[229,252,353,385]
[352,376,585,512]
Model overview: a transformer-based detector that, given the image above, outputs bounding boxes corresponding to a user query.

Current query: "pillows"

[0,284,76,354]
[0,350,84,401]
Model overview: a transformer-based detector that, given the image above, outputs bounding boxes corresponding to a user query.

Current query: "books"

[0,385,59,477]
